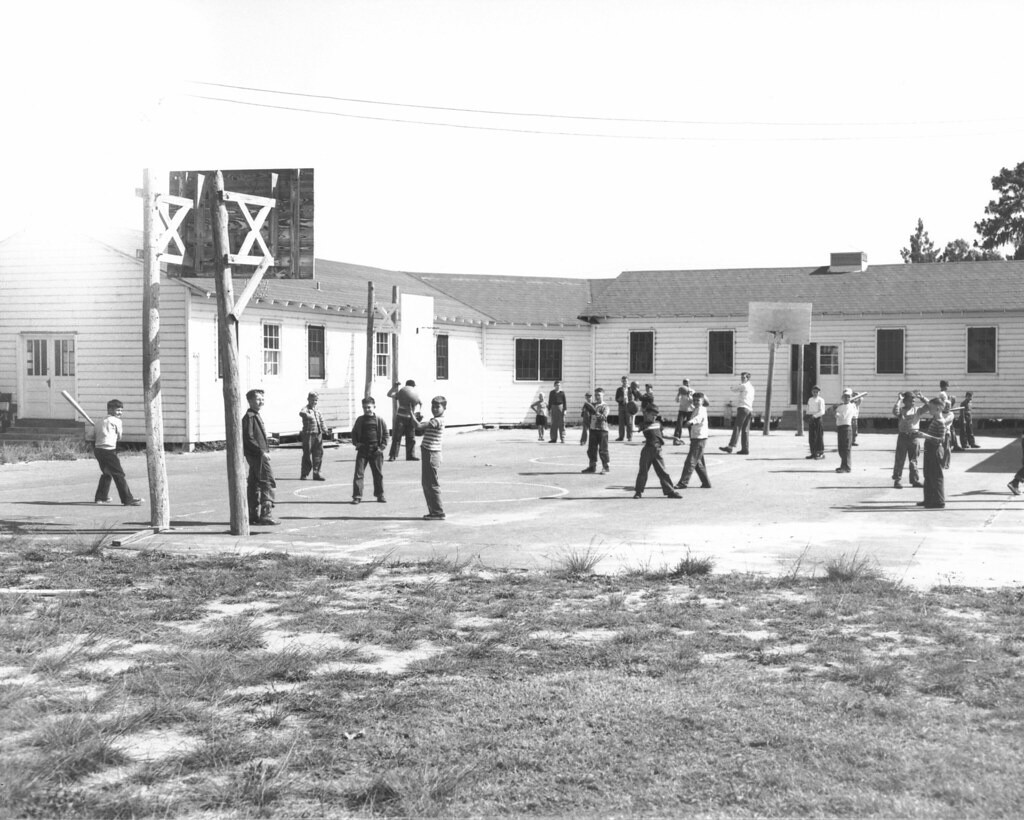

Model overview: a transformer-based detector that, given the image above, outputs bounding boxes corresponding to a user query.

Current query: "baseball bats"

[828,391,867,411]
[61,390,95,426]
[951,406,966,412]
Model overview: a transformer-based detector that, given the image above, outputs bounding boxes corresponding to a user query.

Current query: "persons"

[719,372,754,454]
[300,391,328,481]
[410,395,445,520]
[1007,428,1024,495]
[530,382,567,444]
[630,403,683,499]
[351,396,389,504]
[803,385,826,460]
[92,399,143,505]
[892,378,980,509]
[581,387,610,475]
[615,376,654,442]
[387,380,422,461]
[674,380,711,489]
[831,388,863,473]
[242,388,280,525]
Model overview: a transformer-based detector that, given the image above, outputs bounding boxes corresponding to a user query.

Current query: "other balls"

[630,381,640,390]
[398,385,420,409]
[627,400,639,415]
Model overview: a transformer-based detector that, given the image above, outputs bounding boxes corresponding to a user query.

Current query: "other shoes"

[407,456,420,461]
[301,475,307,480]
[581,430,980,473]
[352,498,360,504]
[424,512,446,520]
[260,517,281,525]
[1008,482,1021,494]
[97,497,112,505]
[549,439,556,443]
[893,481,904,489]
[378,496,387,502]
[604,463,610,472]
[917,501,945,509]
[314,475,325,481]
[912,482,924,487]
[249,515,261,526]
[582,468,595,473]
[673,482,687,489]
[634,492,641,499]
[700,484,707,488]
[667,492,682,499]
[125,498,144,506]
[390,455,396,461]
